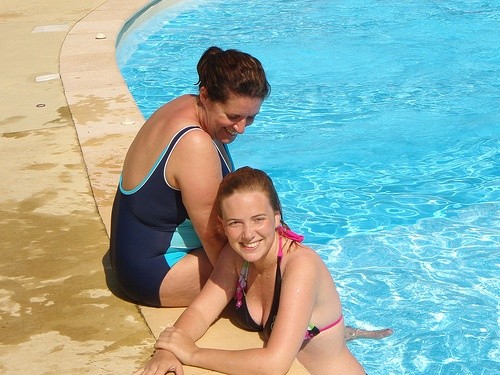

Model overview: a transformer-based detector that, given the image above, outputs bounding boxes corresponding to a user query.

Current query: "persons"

[109,44,393,342]
[132,163,369,375]
